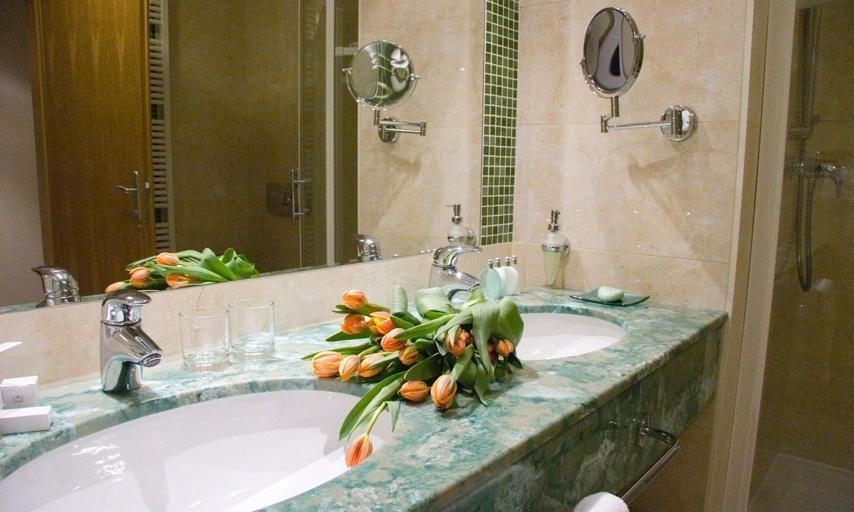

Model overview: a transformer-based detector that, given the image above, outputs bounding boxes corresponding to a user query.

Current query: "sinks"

[0,377,401,512]
[498,303,629,361]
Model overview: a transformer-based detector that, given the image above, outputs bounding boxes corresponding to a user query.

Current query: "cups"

[177,297,275,372]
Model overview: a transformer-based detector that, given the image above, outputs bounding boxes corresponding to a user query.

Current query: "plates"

[569,288,650,306]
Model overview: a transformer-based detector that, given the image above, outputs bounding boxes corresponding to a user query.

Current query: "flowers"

[301,285,524,469]
[103,248,257,294]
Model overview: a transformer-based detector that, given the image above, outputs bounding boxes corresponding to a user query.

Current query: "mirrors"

[577,8,696,144]
[0,0,483,313]
[341,39,428,144]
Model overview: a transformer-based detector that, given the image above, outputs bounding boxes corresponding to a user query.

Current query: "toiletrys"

[479,255,525,301]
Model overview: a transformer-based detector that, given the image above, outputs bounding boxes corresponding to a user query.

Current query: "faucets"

[428,245,484,298]
[350,234,382,262]
[29,265,81,306]
[99,287,163,393]
